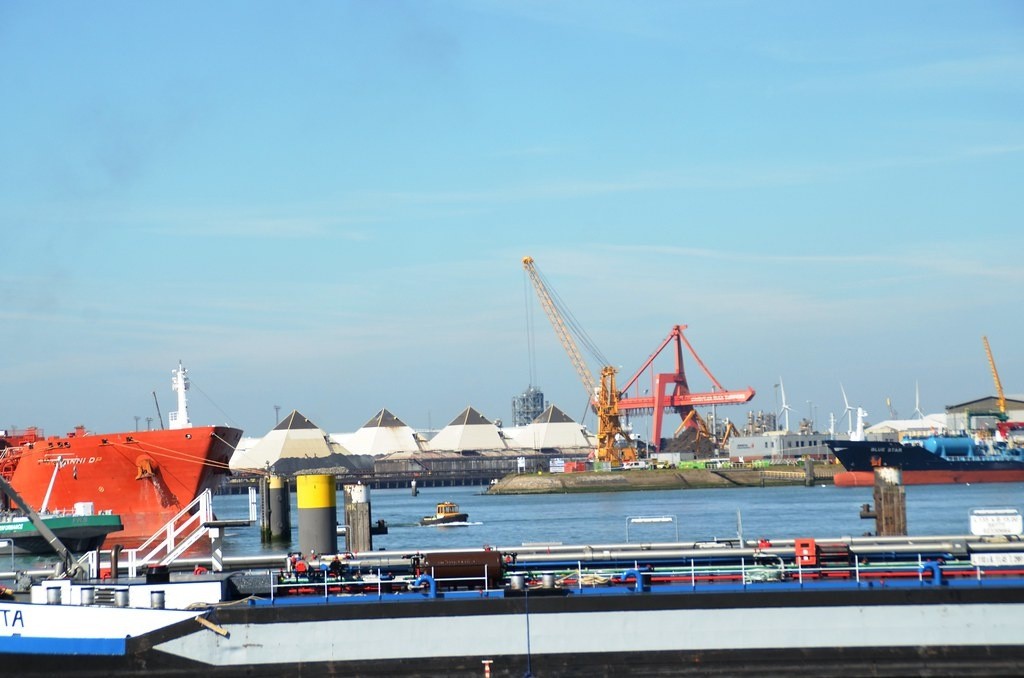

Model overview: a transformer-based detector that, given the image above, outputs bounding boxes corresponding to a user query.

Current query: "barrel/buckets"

[151,590,165,609]
[114,589,129,607]
[80,587,95,605]
[47,586,62,605]
[509,573,525,590]
[541,573,554,588]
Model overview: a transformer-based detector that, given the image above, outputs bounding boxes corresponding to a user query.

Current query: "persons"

[0,505,60,517]
[288,550,364,593]
[101,510,104,515]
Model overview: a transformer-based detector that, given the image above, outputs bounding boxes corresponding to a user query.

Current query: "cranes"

[981,335,1008,423]
[521,256,637,469]
[591,325,755,453]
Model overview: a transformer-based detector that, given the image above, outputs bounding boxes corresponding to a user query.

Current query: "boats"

[819,438,1024,486]
[419,502,470,526]
[0,358,243,559]
